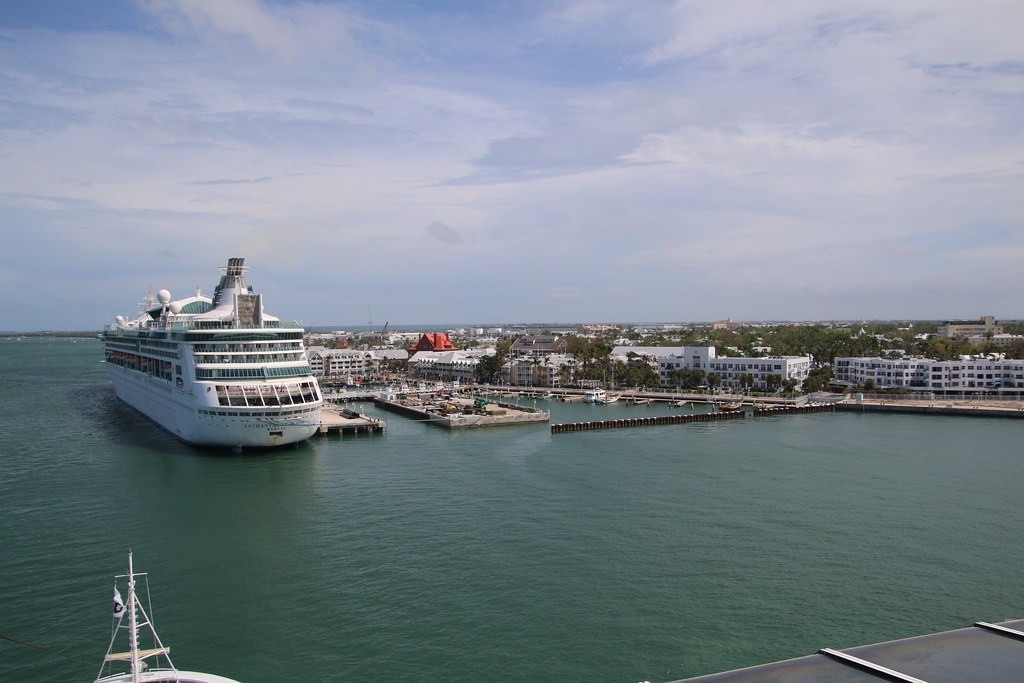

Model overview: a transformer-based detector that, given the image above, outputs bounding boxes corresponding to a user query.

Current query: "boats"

[539,390,553,400]
[582,387,606,403]
[84,548,247,683]
[719,401,744,410]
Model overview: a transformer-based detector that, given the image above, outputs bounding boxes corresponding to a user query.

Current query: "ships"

[103,257,323,448]
